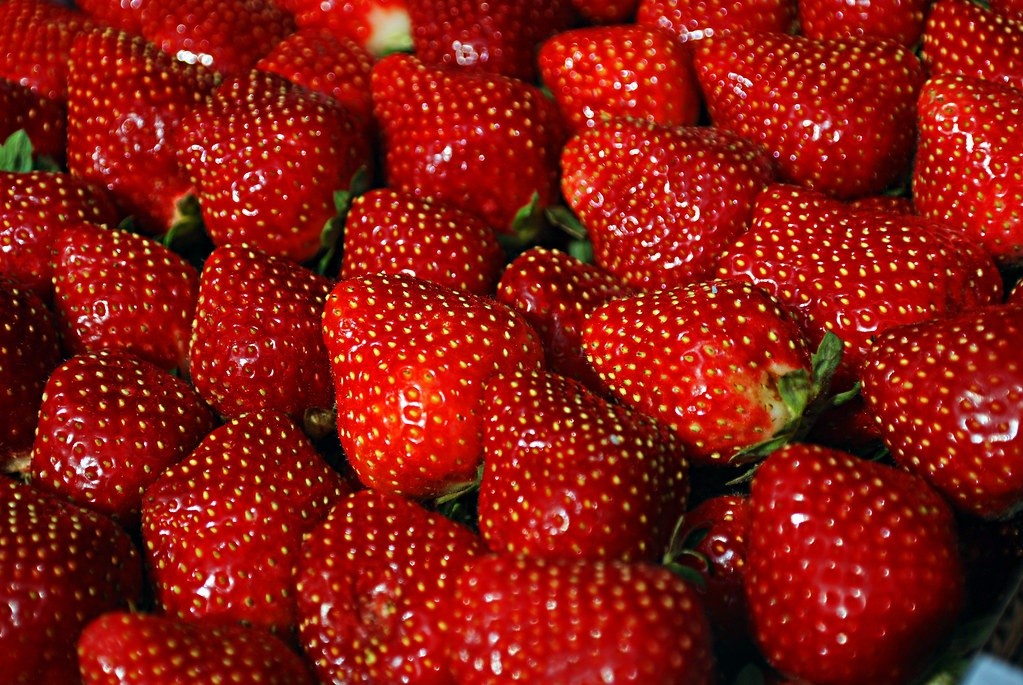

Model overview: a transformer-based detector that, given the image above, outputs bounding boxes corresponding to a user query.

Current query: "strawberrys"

[0,0,1023,685]
